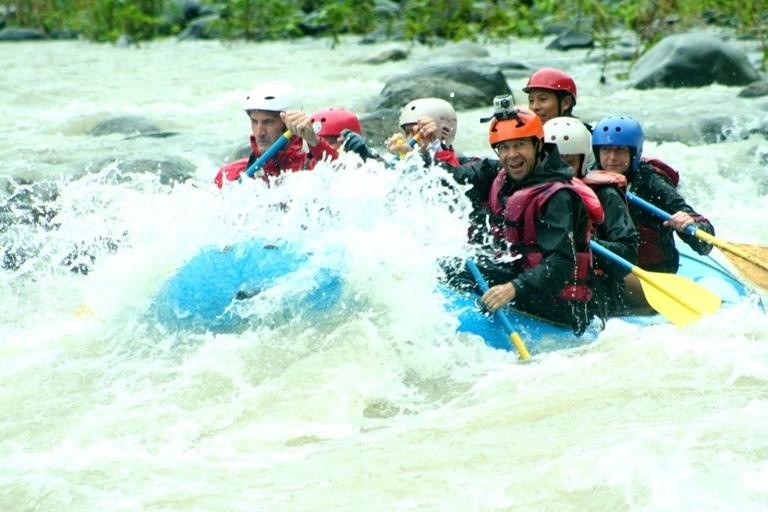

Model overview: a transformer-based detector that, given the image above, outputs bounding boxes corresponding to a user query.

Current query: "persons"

[384,103,607,338]
[304,106,392,170]
[385,97,482,169]
[210,82,339,190]
[540,114,643,321]
[591,113,716,315]
[521,67,595,138]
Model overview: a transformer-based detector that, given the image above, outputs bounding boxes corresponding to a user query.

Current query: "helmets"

[591,114,643,175]
[541,116,589,177]
[310,106,361,137]
[521,67,576,105]
[398,97,456,150]
[488,105,543,145]
[243,81,304,114]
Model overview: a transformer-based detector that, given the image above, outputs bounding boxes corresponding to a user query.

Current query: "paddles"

[626,191,768,289]
[591,239,720,324]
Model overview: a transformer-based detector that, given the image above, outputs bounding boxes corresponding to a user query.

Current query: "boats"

[52,229,767,393]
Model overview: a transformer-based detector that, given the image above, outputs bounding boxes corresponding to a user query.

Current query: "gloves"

[340,127,372,160]
